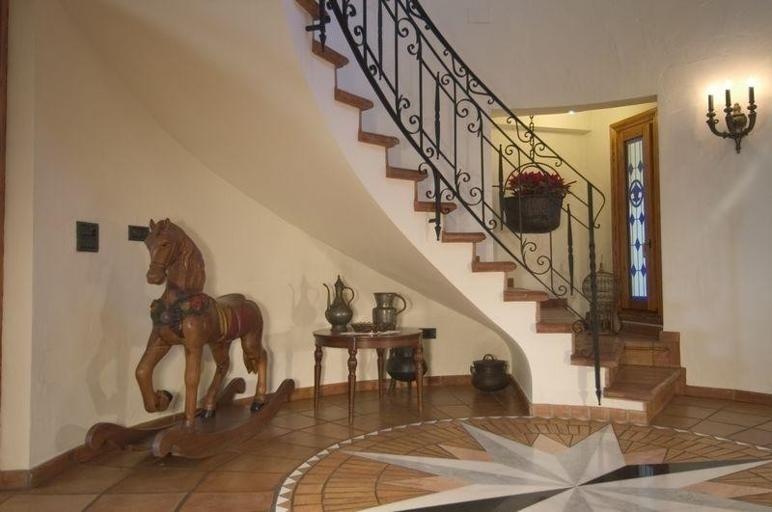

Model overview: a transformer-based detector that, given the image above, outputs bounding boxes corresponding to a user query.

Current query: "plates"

[349,322,392,333]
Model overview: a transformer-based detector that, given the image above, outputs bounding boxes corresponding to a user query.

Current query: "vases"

[501,192,564,233]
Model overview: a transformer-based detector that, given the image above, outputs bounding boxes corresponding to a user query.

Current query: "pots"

[470,353,509,392]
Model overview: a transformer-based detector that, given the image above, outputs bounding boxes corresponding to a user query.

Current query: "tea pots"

[323,274,355,332]
[372,292,406,331]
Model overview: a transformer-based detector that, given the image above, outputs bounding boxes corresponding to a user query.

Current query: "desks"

[307,324,426,425]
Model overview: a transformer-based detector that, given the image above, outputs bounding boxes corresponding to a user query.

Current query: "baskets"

[502,162,568,233]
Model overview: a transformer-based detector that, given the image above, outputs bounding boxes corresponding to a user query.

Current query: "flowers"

[495,172,572,199]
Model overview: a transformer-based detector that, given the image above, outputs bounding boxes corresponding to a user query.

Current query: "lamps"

[702,82,764,156]
